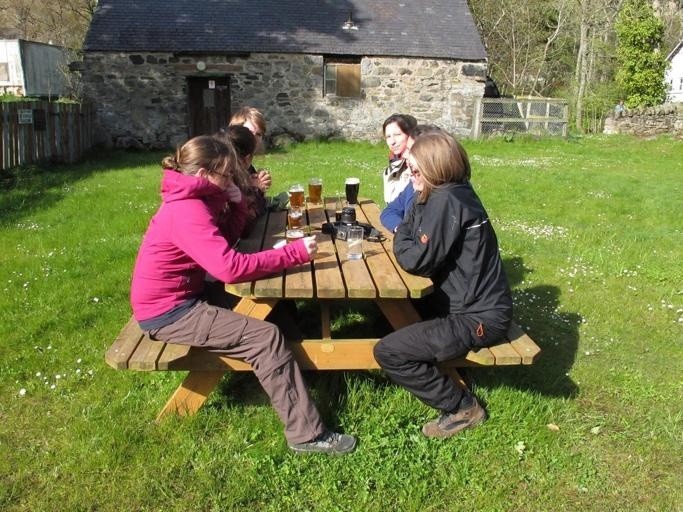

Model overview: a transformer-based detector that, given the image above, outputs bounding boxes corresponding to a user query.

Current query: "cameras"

[323,206,373,240]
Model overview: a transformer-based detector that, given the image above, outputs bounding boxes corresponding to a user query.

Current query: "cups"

[345,177,360,204]
[288,183,304,207]
[287,207,305,230]
[346,226,364,259]
[308,178,322,202]
[286,229,304,246]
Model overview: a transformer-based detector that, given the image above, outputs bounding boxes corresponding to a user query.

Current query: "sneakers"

[421,395,486,437]
[287,430,357,452]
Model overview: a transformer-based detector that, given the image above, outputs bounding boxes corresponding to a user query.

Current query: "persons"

[128,133,359,456]
[382,113,418,207]
[614,99,624,110]
[225,105,269,241]
[371,128,513,438]
[202,124,272,287]
[378,123,450,237]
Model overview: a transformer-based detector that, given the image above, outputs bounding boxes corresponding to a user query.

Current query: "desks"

[153,196,466,424]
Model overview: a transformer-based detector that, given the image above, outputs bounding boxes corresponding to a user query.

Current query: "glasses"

[208,169,231,179]
